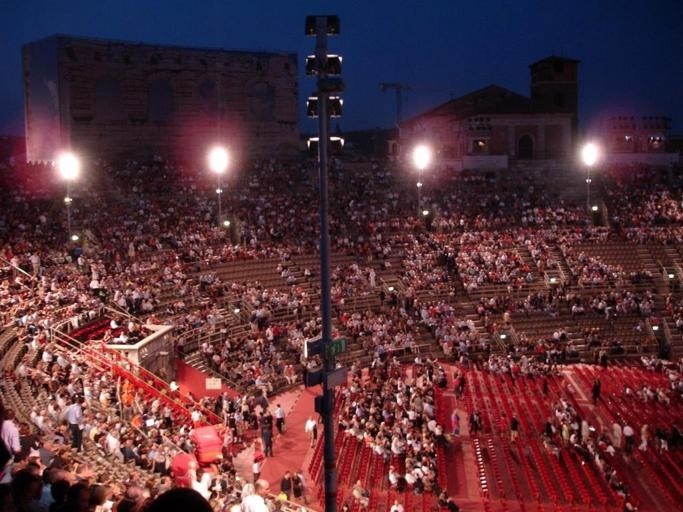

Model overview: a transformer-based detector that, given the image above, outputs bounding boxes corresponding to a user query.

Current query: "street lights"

[208,147,228,229]
[302,17,346,512]
[581,143,597,215]
[413,145,428,218]
[57,153,80,242]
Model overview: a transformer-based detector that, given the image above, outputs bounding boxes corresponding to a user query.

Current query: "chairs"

[0,244,683,512]
